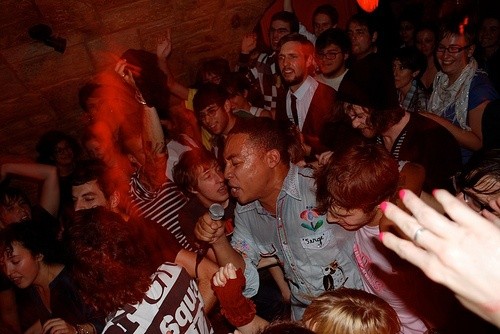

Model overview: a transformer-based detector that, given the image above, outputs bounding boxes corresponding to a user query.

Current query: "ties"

[289,94,300,132]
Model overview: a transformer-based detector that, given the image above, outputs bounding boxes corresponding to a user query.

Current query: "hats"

[336,54,401,108]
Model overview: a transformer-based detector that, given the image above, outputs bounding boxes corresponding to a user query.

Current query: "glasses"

[317,51,342,60]
[436,44,470,53]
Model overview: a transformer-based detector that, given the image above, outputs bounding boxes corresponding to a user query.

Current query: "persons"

[0,0,500,334]
[377,189,500,327]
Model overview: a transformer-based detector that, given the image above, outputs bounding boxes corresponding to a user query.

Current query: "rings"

[122,74,125,78]
[413,228,426,242]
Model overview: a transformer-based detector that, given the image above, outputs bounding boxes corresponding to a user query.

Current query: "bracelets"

[74,325,89,334]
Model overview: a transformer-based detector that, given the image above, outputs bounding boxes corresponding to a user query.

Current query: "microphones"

[196,204,225,264]
